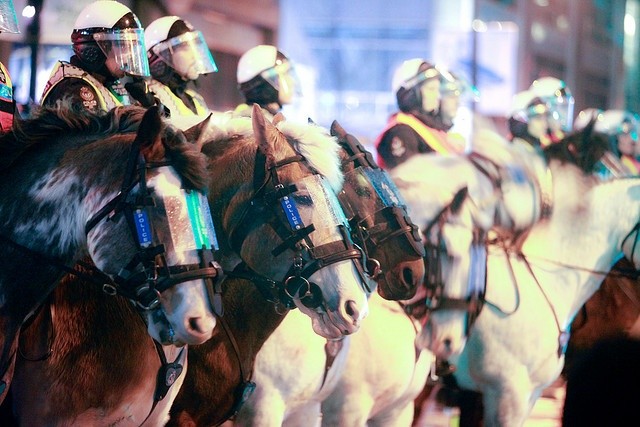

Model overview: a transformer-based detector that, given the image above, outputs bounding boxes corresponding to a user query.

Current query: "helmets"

[71,0,150,76]
[438,62,481,104]
[594,109,637,152]
[237,45,304,105]
[529,76,575,131]
[144,15,218,77]
[509,91,549,136]
[392,59,457,113]
[574,108,601,133]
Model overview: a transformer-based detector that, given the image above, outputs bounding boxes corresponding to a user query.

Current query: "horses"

[0,97,225,427]
[164,101,371,427]
[427,118,640,427]
[413,256,640,427]
[322,151,499,427]
[217,116,427,427]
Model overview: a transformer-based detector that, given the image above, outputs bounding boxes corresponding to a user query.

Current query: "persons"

[628,113,640,173]
[374,56,456,170]
[1,0,21,132]
[574,108,616,181]
[507,90,563,192]
[530,75,575,142]
[604,108,639,177]
[225,43,306,131]
[143,16,220,125]
[434,64,482,131]
[38,1,151,115]
